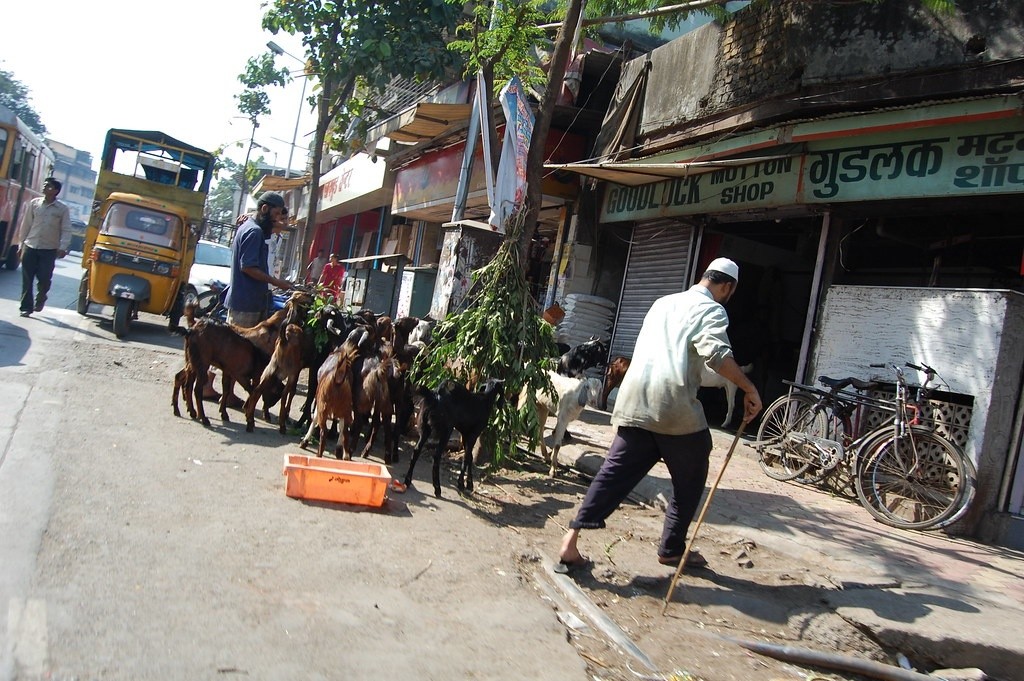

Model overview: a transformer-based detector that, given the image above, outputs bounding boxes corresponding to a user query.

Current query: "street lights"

[266,41,310,278]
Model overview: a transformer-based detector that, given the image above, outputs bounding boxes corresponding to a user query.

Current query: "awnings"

[367,102,471,159]
[251,174,308,200]
[235,212,288,234]
[542,155,792,188]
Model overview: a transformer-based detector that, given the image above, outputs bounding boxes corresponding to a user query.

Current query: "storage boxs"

[280,452,395,509]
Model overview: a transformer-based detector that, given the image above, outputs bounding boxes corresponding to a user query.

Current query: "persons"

[553,257,763,574]
[310,249,345,305]
[201,191,293,409]
[17,178,72,317]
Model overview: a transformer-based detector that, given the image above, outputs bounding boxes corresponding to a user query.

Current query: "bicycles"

[742,362,979,532]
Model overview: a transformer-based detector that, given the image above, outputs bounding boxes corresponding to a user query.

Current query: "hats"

[706,257,739,282]
[259,190,285,207]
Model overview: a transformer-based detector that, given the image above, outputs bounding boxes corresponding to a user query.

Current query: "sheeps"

[167,290,754,499]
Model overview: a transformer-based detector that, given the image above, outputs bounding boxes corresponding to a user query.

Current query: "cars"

[182,240,232,318]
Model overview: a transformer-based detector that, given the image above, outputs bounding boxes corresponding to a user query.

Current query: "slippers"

[662,550,707,566]
[218,393,244,408]
[202,391,222,401]
[553,555,591,574]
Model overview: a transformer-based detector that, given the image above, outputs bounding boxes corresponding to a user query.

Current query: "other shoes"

[20,309,30,317]
[33,300,45,312]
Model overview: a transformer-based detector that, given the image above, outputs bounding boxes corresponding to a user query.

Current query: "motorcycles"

[70,218,88,253]
[76,128,217,341]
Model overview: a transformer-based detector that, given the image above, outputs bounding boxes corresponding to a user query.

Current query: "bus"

[0,105,55,271]
